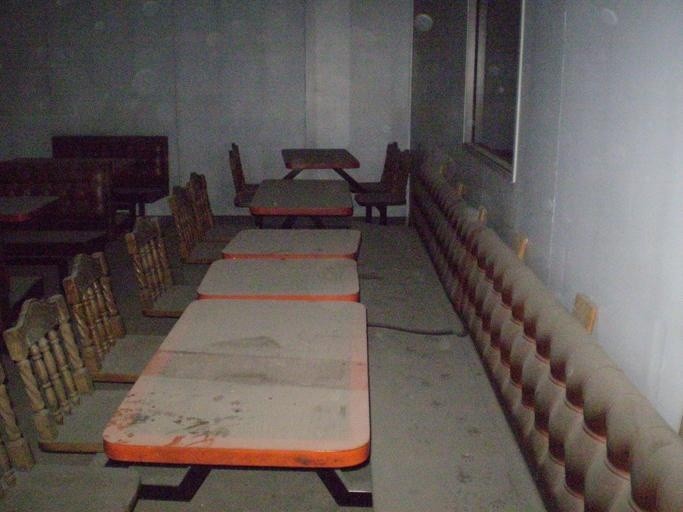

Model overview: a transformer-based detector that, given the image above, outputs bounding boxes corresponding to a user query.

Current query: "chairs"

[1,174,375,512]
[224,142,408,228]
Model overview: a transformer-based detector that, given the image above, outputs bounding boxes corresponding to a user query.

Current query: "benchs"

[357,159,682,512]
[0,137,169,290]
